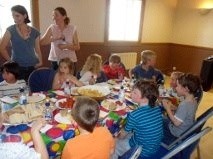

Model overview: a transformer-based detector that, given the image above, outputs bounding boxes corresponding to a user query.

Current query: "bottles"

[170,67,178,89]
[62,38,68,50]
[63,81,71,99]
[118,86,125,102]
[42,102,53,124]
[18,89,27,106]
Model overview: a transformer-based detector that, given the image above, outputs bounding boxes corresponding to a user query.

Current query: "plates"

[101,98,127,111]
[54,109,73,124]
[26,93,47,103]
[55,98,68,109]
[78,85,111,97]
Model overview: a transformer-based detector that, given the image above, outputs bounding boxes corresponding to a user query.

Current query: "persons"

[118,80,163,159]
[0,102,49,159]
[163,72,203,144]
[60,96,116,159]
[79,54,102,85]
[0,62,31,99]
[133,50,164,83]
[53,58,82,89]
[102,55,126,81]
[0,5,42,79]
[41,7,80,74]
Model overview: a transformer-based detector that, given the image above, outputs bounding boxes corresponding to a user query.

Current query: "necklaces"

[18,24,31,40]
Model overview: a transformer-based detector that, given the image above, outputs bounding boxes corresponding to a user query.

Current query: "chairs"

[104,61,125,82]
[118,144,142,159]
[28,67,56,93]
[160,127,213,159]
[75,70,107,84]
[160,106,213,150]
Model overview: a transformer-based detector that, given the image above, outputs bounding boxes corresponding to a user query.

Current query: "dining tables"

[0,78,185,159]
